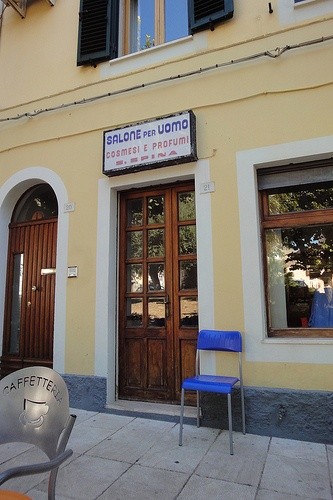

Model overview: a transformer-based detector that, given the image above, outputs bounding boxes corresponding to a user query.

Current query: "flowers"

[143,34,155,49]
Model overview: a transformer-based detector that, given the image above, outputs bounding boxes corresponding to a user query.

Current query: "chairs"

[1,365,77,500]
[178,330,245,457]
